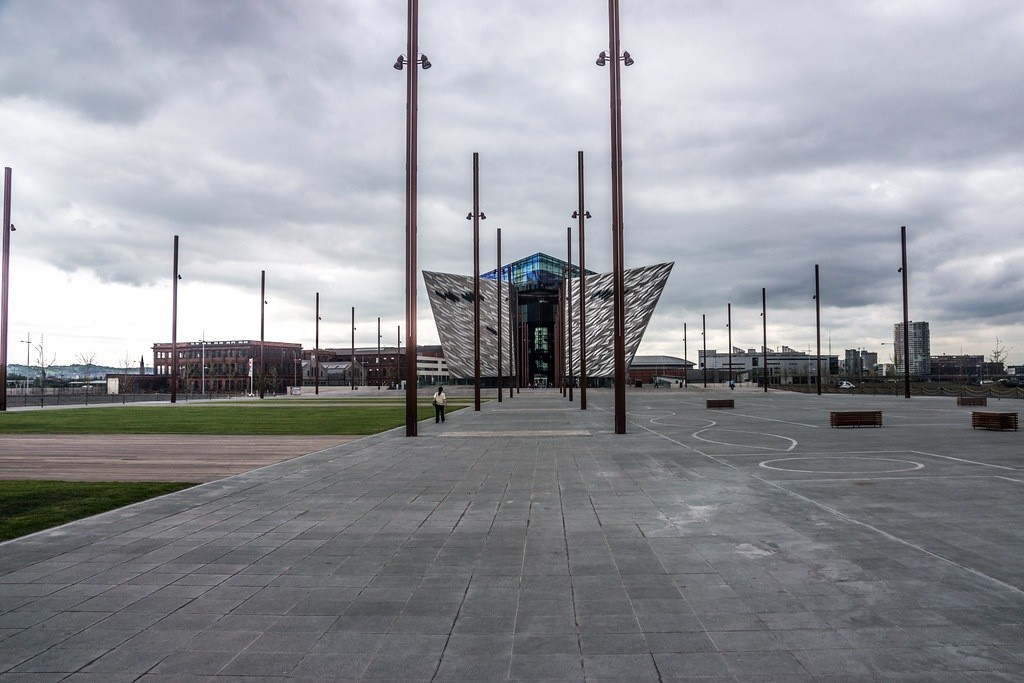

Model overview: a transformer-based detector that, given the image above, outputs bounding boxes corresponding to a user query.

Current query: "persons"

[730,378,734,389]
[654,377,659,389]
[432,387,448,424]
[527,381,553,389]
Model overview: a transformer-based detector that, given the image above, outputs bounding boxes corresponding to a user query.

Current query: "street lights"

[898,267,910,397]
[726,324,731,387]
[813,295,821,395]
[395,54,430,436]
[506,298,516,397]
[493,268,505,402]
[377,334,382,389]
[352,327,357,390]
[171,274,182,401]
[259,300,268,397]
[0,221,16,410]
[466,213,488,410]
[597,51,633,428]
[761,312,767,392]
[397,341,403,390]
[198,339,205,395]
[564,269,576,400]
[702,332,706,388]
[21,340,31,394]
[683,338,687,389]
[572,211,592,409]
[315,317,321,393]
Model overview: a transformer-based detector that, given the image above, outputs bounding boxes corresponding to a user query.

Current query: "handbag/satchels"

[432,398,436,406]
